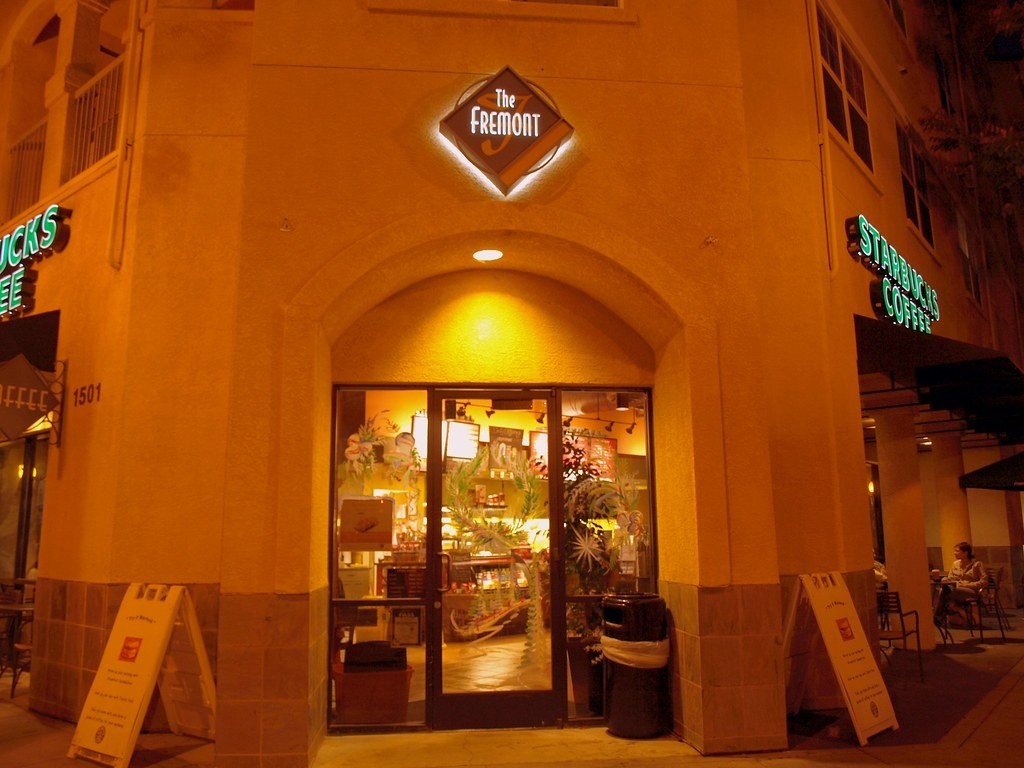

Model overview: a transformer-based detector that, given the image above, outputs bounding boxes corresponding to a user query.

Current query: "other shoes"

[934,615,943,627]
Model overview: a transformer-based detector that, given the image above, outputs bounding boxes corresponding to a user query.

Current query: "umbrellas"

[959,451,1024,492]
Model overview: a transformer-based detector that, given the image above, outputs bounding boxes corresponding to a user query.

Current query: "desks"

[0,603,34,699]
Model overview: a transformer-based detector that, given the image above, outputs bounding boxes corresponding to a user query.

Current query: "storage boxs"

[332,663,414,724]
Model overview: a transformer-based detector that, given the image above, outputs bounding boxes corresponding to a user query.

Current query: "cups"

[932,569,940,579]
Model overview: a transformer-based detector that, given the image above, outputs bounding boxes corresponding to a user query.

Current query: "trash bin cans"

[601,593,672,740]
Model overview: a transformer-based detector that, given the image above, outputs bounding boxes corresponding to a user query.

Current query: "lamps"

[605,421,614,431]
[486,407,495,418]
[564,416,573,426]
[626,424,636,434]
[536,412,545,423]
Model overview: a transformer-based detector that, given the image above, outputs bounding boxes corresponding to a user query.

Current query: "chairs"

[945,566,1011,643]
[878,591,923,683]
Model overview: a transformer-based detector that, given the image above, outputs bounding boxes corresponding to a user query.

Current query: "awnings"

[0,309,60,372]
[853,313,1024,448]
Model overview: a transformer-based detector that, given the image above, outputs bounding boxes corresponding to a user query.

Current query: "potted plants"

[559,438,624,718]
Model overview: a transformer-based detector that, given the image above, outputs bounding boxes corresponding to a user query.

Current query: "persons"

[929,542,989,627]
[872,545,888,591]
